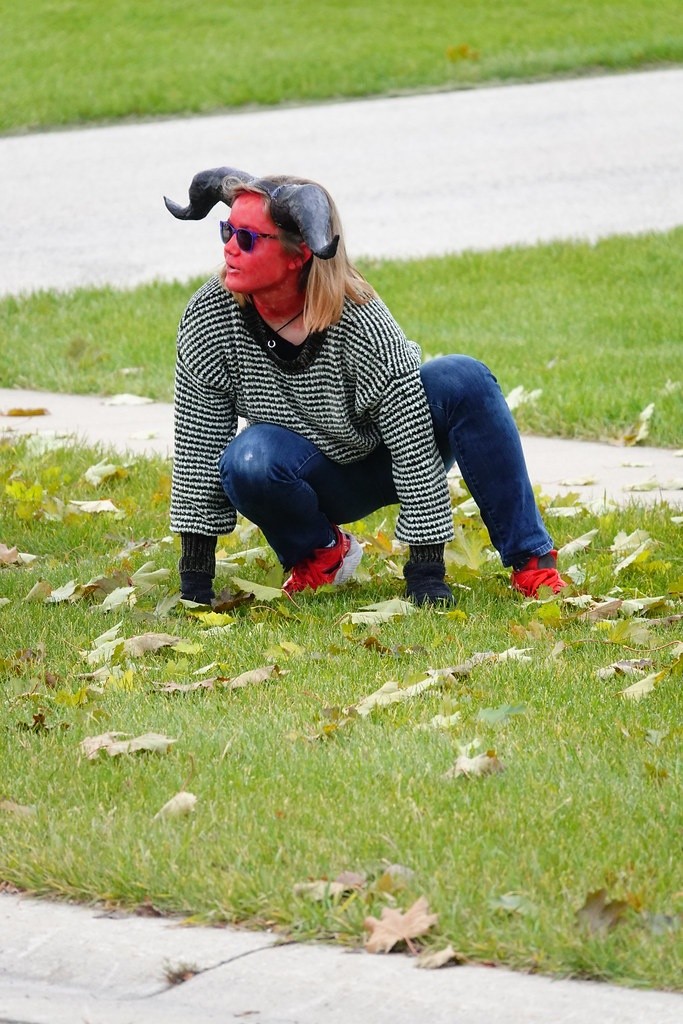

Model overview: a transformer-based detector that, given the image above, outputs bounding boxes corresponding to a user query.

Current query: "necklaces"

[252,292,303,347]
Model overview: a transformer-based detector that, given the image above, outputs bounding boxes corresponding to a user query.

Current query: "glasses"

[220,221,283,252]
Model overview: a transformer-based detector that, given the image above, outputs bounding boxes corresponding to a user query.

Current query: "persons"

[174,175,573,614]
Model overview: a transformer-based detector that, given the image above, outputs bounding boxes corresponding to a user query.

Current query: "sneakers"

[282,526,363,597]
[511,550,569,601]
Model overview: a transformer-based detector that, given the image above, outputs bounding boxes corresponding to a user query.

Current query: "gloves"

[179,573,215,607]
[404,564,457,610]
